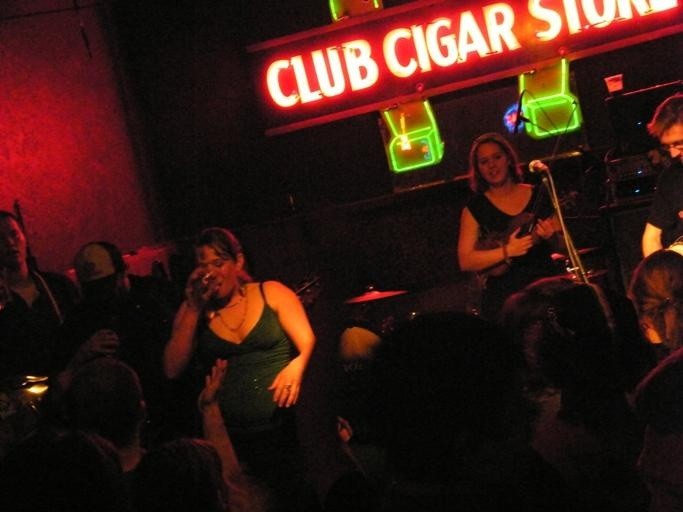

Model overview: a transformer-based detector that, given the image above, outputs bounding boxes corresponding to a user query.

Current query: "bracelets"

[501,247,510,260]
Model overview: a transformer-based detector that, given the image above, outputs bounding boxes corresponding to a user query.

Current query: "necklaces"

[210,284,252,333]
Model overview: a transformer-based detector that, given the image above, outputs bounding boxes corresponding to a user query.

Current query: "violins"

[476,190,579,278]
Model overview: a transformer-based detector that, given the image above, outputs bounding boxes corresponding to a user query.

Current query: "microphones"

[528,159,547,177]
[514,92,524,136]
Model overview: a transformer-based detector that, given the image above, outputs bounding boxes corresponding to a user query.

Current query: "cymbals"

[343,290,411,305]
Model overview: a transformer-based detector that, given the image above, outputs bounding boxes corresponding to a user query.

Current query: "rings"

[283,381,294,392]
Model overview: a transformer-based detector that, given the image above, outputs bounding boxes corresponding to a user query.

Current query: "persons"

[74,236,179,352]
[65,354,262,512]
[641,94,682,264]
[457,131,563,290]
[0,211,75,424]
[624,248,681,512]
[329,297,642,512]
[163,227,318,426]
[506,275,645,511]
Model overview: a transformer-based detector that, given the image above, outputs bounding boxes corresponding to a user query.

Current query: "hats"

[74,241,124,306]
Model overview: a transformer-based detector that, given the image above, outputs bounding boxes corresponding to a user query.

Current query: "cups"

[190,269,223,300]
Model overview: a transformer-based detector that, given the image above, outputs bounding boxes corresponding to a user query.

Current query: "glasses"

[658,140,683,150]
